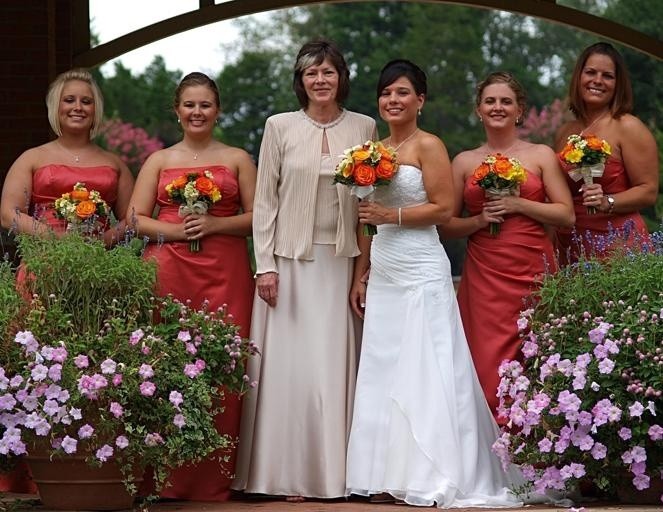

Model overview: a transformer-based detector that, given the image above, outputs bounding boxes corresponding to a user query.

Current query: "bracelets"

[398,207,402,228]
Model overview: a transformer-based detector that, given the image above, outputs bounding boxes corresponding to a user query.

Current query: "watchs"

[604,195,615,215]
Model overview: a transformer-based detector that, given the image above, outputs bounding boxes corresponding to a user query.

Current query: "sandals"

[368,492,408,505]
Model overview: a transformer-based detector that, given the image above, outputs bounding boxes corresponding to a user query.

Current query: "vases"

[23,420,144,511]
[617,456,659,502]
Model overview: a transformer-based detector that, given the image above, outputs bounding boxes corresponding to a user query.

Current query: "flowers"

[489,216,663,502]
[330,139,400,238]
[54,180,116,237]
[165,168,222,253]
[472,151,529,199]
[0,291,263,512]
[557,131,612,215]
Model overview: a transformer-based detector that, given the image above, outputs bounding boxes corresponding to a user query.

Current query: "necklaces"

[579,107,609,137]
[389,128,420,151]
[55,139,89,163]
[193,153,199,160]
[485,142,517,155]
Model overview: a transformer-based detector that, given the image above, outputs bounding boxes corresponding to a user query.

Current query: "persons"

[229,41,380,503]
[554,42,660,268]
[0,68,135,496]
[126,71,258,503]
[437,73,577,469]
[344,59,575,510]
